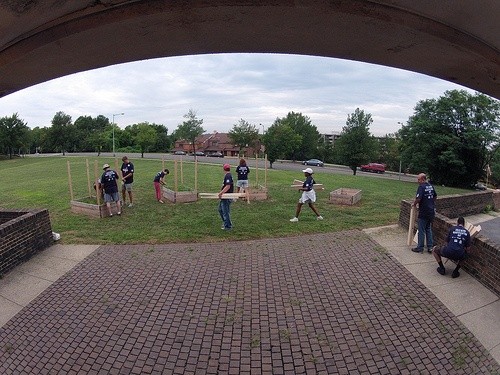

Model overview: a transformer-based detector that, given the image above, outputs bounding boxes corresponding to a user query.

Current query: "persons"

[411,173,437,253]
[233,158,250,205]
[218,163,234,230]
[351,159,357,175]
[93,164,122,217]
[432,217,471,278]
[289,167,323,222]
[153,168,169,203]
[120,155,134,207]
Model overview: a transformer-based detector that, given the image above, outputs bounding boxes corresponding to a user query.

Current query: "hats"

[103,163,110,170]
[458,217,464,222]
[302,168,313,174]
[224,163,230,171]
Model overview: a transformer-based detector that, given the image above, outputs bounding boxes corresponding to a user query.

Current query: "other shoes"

[452,271,460,278]
[428,248,433,253]
[109,213,112,217]
[117,211,120,214]
[128,202,133,207]
[221,224,233,232]
[412,248,423,252]
[159,199,164,204]
[437,267,445,275]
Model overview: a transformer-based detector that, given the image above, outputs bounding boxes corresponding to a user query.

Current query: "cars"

[192,152,205,156]
[302,158,324,167]
[472,183,488,191]
[173,151,186,155]
[206,152,224,158]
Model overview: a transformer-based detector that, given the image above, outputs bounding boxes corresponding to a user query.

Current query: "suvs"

[361,163,386,174]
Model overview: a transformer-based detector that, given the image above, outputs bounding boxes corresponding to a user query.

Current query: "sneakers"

[316,215,323,221]
[290,216,299,222]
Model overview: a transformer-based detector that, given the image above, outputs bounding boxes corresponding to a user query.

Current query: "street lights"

[112,113,125,157]
[259,123,264,135]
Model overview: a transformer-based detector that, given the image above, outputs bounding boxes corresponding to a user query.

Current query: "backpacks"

[419,198,435,219]
